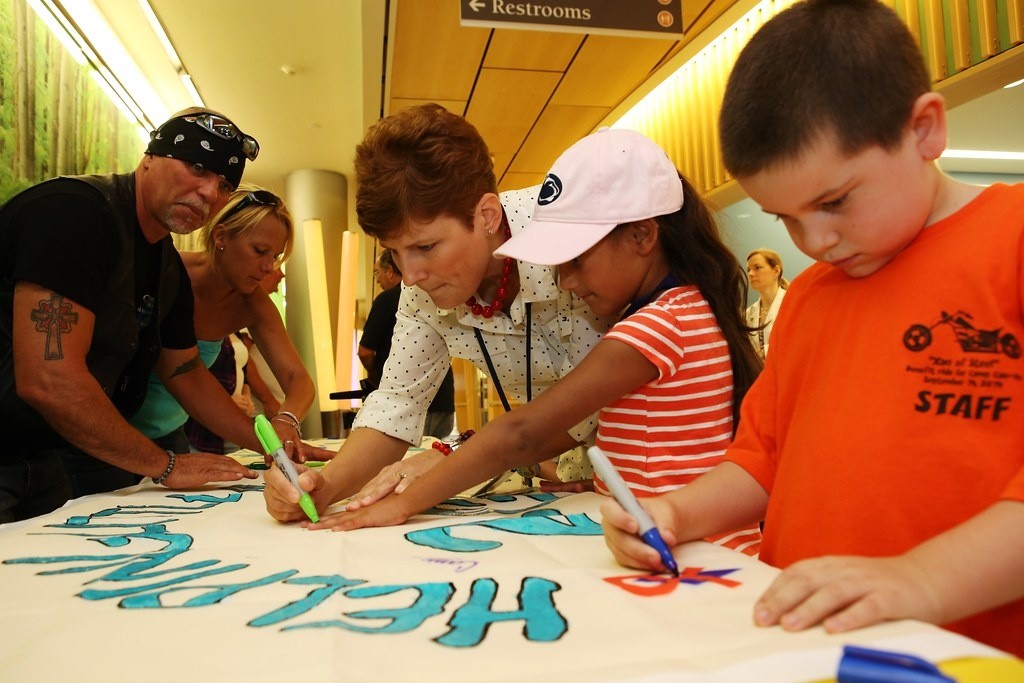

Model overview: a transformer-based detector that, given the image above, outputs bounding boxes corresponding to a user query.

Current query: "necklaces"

[465,212,512,318]
[760,307,769,315]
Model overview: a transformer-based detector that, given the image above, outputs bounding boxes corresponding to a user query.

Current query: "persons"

[0,0,1024,663]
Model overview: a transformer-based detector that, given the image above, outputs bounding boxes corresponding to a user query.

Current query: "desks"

[0,440,1024,683]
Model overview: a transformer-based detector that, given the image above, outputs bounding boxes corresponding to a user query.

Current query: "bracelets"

[152,450,176,484]
[460,430,475,442]
[432,442,451,457]
[270,418,303,438]
[278,411,299,425]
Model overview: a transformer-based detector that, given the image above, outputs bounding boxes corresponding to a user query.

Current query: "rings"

[285,441,294,444]
[393,473,407,481]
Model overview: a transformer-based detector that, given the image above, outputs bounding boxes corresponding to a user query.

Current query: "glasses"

[160,112,260,162]
[215,190,283,225]
[373,267,388,278]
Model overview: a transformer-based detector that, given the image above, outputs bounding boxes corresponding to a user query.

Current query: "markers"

[252,412,318,524]
[585,441,681,579]
[299,459,328,467]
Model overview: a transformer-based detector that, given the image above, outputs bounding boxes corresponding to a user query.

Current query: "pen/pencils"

[248,461,273,470]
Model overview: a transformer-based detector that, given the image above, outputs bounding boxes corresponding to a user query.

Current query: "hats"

[492,126,684,267]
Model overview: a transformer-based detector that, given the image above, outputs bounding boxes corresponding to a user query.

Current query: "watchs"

[441,434,461,452]
